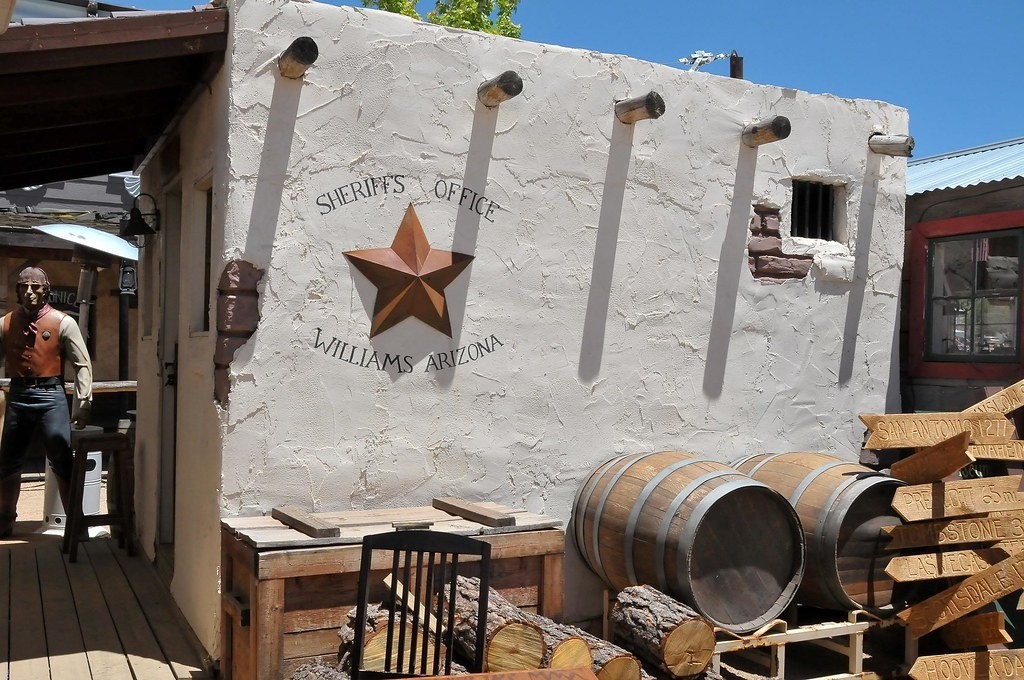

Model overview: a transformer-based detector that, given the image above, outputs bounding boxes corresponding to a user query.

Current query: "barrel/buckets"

[570,447,926,636]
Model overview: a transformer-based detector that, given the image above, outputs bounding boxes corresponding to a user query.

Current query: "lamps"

[120,193,161,237]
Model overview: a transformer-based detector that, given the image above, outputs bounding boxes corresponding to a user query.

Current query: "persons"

[0,267,93,540]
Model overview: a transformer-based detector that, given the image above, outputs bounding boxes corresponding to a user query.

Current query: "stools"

[62,433,134,561]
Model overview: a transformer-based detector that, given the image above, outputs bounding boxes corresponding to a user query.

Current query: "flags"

[971,238,989,262]
